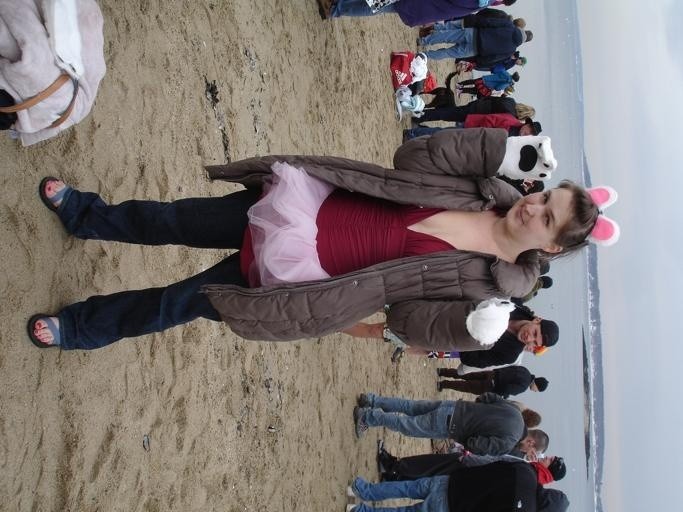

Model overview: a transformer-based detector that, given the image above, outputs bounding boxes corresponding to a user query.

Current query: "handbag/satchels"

[390,51,428,91]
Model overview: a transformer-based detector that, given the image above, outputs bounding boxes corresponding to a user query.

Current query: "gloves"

[498,135,557,182]
[466,298,515,346]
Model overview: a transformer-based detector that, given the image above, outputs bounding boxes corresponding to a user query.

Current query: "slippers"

[26,313,62,349]
[39,175,71,211]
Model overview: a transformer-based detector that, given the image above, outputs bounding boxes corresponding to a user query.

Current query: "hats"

[546,455,566,480]
[522,57,527,66]
[534,377,549,392]
[525,117,542,136]
[541,319,559,348]
[540,276,552,289]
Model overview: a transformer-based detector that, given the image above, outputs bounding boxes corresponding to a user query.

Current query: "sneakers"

[353,406,368,439]
[437,382,443,392]
[378,439,392,473]
[358,393,368,408]
[437,367,441,381]
[318,0,336,21]
